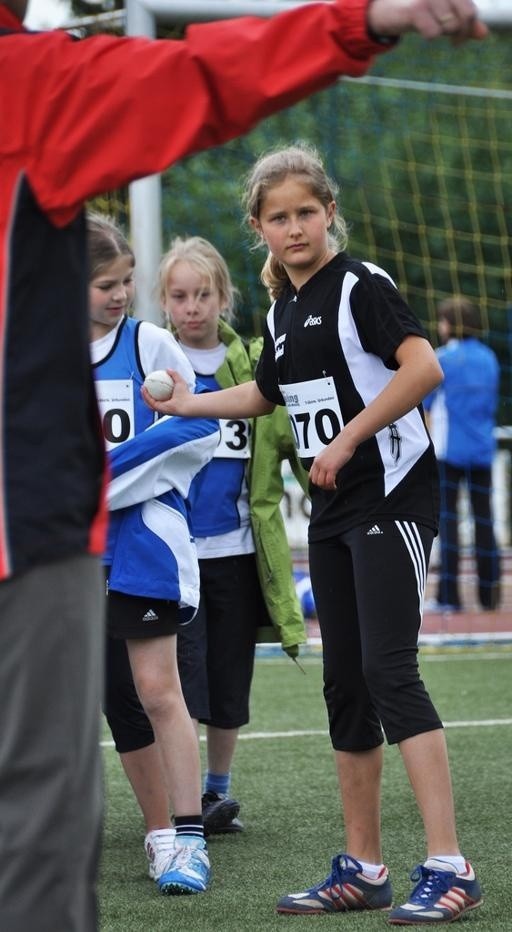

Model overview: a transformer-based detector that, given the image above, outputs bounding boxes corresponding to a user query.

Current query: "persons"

[420,296,502,616]
[150,234,312,837]
[2,0,491,932]
[86,213,222,895]
[139,146,484,925]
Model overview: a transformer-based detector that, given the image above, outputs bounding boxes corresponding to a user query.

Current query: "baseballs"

[145,371,174,402]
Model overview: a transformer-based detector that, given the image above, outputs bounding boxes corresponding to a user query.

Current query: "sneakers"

[389,856,485,925]
[201,792,247,837]
[426,599,460,612]
[273,853,393,914]
[145,829,212,896]
